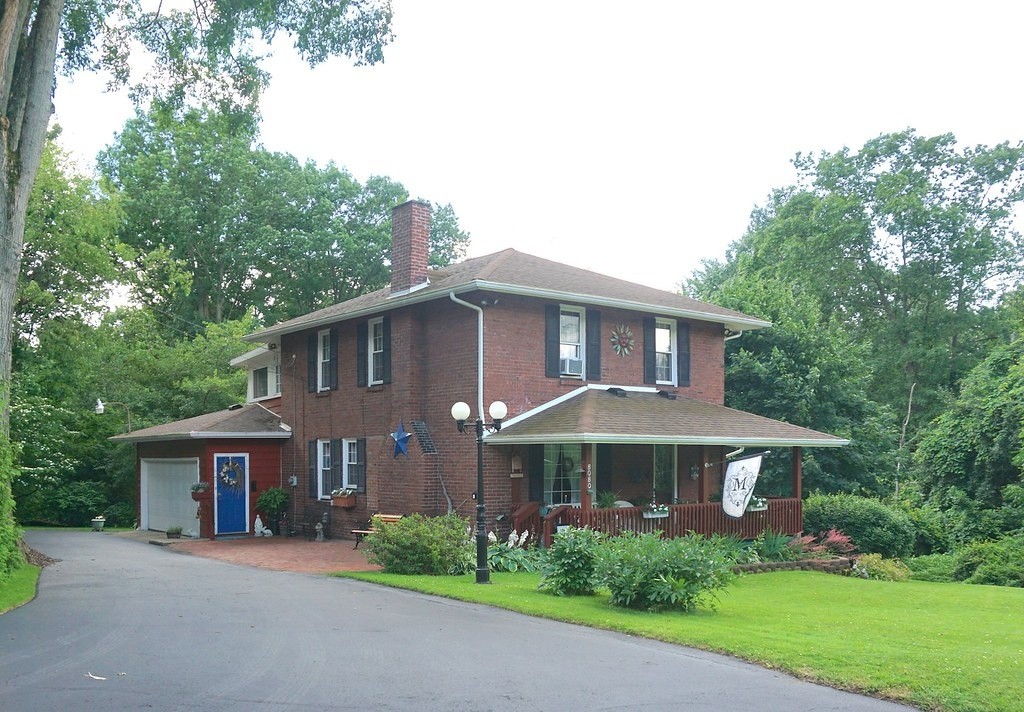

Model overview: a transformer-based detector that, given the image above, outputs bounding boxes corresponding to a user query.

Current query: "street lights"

[451,401,508,584]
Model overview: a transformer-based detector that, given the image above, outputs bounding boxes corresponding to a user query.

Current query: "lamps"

[490,296,499,304]
[480,297,489,305]
[271,343,276,349]
[724,329,734,337]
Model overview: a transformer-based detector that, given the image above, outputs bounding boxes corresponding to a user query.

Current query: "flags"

[721,453,763,519]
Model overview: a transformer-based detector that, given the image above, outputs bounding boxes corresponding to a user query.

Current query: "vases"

[746,506,768,511]
[643,512,668,519]
[334,495,356,507]
[192,491,210,502]
[91,519,106,529]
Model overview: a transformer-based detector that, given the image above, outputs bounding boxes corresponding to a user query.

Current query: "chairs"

[613,501,635,528]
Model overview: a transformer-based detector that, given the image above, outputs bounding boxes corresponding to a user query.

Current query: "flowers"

[95,515,106,519]
[188,481,211,493]
[642,502,670,513]
[332,488,353,496]
[746,495,767,507]
[220,462,243,489]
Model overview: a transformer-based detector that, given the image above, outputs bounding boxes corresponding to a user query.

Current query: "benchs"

[293,505,332,542]
[350,512,407,550]
[555,503,599,523]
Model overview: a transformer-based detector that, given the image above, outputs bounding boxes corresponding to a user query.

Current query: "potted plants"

[166,525,185,538]
[255,487,289,536]
